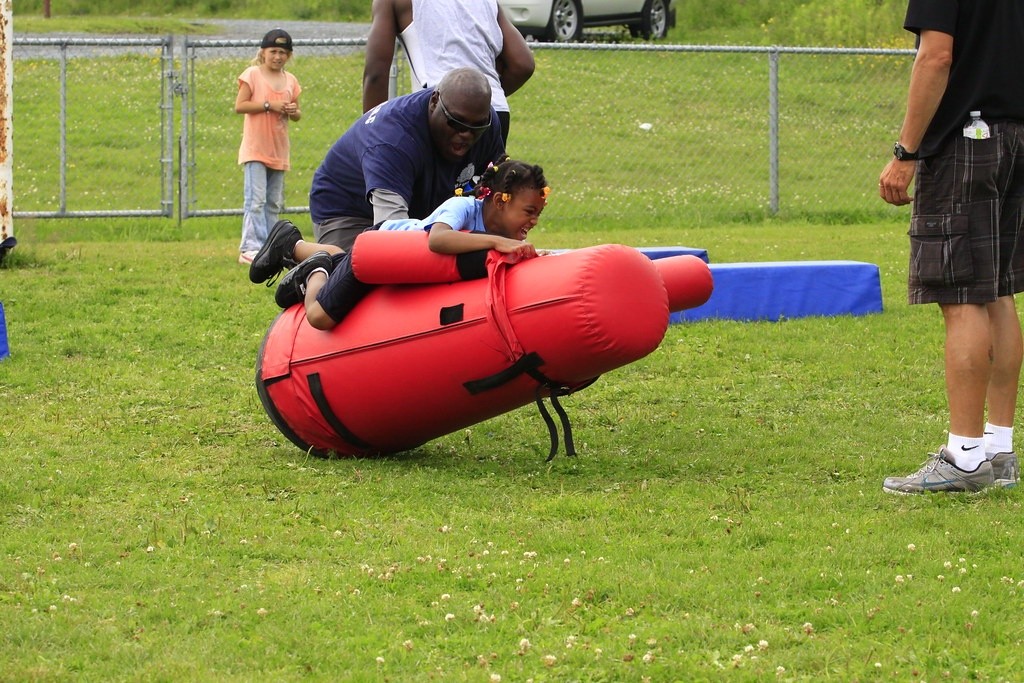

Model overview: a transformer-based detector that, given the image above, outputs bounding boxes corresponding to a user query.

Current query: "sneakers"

[238,250,260,265]
[986,449,1021,493]
[880,444,994,499]
[247,219,299,284]
[274,250,332,309]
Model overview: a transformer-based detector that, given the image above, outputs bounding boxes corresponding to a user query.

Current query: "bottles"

[962,111,990,139]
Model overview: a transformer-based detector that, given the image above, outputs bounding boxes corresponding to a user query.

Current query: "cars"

[498,0,670,44]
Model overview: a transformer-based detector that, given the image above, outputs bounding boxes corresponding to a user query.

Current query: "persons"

[877,0,1024,498]
[234,27,304,264]
[309,68,504,254]
[363,0,536,150]
[248,154,552,331]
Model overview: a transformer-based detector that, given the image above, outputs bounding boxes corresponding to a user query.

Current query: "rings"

[878,183,884,187]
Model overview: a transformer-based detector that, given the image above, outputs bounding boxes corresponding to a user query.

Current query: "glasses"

[438,94,492,137]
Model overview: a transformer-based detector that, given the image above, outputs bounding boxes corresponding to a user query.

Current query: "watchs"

[893,142,920,161]
[264,101,270,111]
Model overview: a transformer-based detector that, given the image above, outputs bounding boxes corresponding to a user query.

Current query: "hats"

[261,28,293,52]
